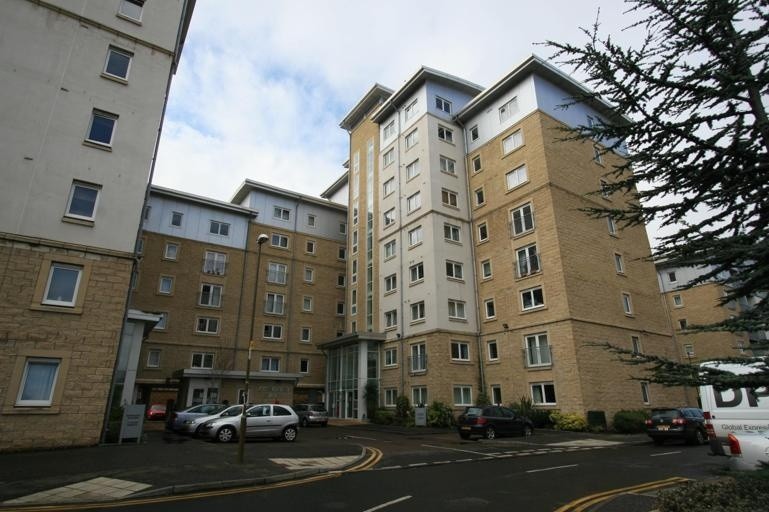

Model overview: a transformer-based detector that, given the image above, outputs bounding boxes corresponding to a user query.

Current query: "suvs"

[458,405,536,441]
[295,404,328,427]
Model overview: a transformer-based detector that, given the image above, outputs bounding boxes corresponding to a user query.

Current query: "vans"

[171,402,300,444]
[698,355,769,456]
[643,406,710,447]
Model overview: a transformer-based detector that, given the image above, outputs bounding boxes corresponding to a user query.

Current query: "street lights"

[236,233,270,464]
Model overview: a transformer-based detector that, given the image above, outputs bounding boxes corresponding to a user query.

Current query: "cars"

[147,402,167,422]
[727,423,769,473]
